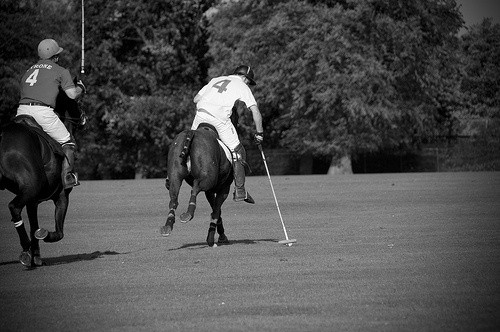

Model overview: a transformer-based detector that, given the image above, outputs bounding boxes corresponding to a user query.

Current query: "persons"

[165,65,264,204]
[15,39,90,190]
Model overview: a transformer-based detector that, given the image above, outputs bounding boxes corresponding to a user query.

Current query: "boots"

[234,160,255,203]
[165,176,170,190]
[61,143,80,188]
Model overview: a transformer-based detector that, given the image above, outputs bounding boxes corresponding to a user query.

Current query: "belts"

[20,102,52,107]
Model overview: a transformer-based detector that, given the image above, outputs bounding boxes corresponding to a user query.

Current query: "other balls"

[286,243,292,247]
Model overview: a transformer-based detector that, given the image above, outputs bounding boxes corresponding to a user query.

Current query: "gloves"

[253,132,263,145]
[78,74,87,94]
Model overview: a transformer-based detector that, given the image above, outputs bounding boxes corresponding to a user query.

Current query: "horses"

[0,75,92,270]
[159,105,240,247]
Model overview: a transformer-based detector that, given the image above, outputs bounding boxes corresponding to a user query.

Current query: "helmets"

[234,65,257,86]
[37,38,63,60]
